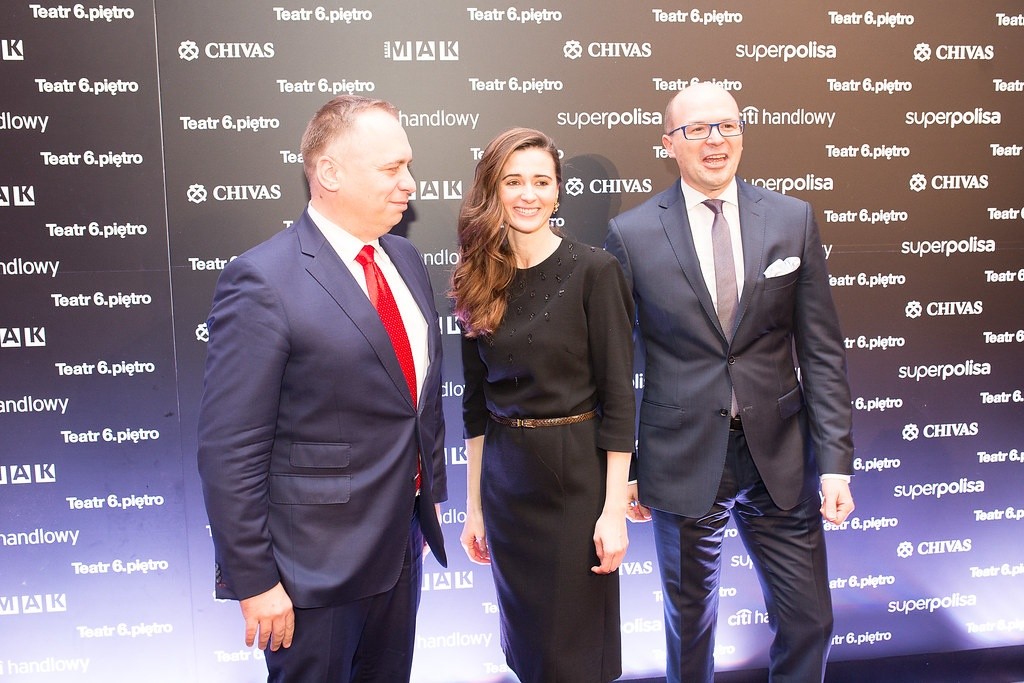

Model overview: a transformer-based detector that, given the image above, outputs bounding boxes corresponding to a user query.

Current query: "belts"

[729,419,743,432]
[488,407,601,428]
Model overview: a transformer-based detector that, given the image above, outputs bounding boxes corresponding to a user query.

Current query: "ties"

[355,245,423,494]
[701,200,740,419]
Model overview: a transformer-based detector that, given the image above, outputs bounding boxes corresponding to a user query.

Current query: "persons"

[438,127,636,683]
[602,81,856,682]
[197,95,453,683]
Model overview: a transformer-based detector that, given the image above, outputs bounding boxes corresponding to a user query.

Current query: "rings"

[475,536,486,544]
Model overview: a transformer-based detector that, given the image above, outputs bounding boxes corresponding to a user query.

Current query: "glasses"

[668,120,745,140]
